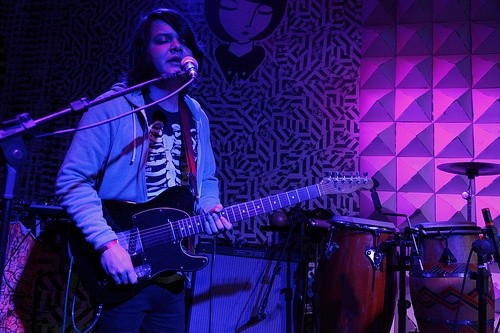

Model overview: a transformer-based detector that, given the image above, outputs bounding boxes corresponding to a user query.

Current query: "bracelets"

[98,239,120,254]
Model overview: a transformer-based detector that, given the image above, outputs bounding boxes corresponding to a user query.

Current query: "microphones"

[180,56,199,79]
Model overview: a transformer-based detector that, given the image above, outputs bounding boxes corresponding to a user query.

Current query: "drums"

[410,222,496,332]
[310,217,401,333]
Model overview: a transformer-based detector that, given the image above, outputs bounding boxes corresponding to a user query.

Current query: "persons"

[53,7,234,333]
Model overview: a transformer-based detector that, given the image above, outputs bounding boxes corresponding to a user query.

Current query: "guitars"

[81,176,374,307]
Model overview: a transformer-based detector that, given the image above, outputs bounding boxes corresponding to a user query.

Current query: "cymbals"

[437,161,500,176]
